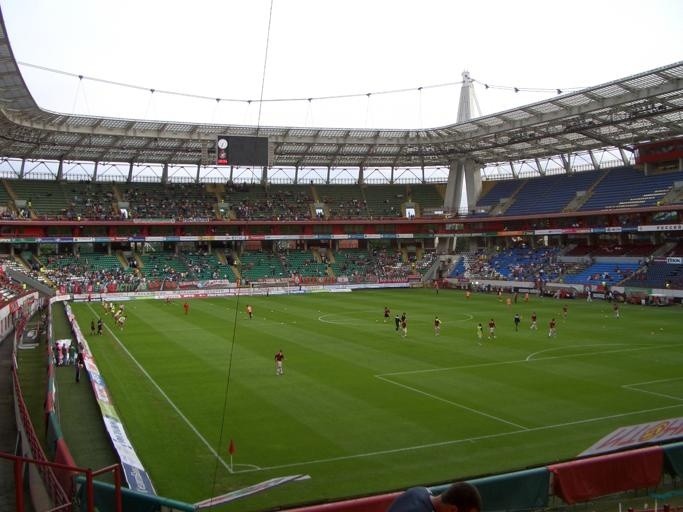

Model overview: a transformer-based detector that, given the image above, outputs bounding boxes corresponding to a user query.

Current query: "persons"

[183,300,190,315]
[86,294,128,336]
[603,290,674,307]
[1,243,683,290]
[52,341,86,382]
[433,279,593,309]
[476,312,558,346]
[0,179,401,222]
[562,304,569,323]
[613,303,620,320]
[434,316,441,337]
[383,303,407,346]
[246,303,254,319]
[386,481,483,512]
[274,349,285,376]
